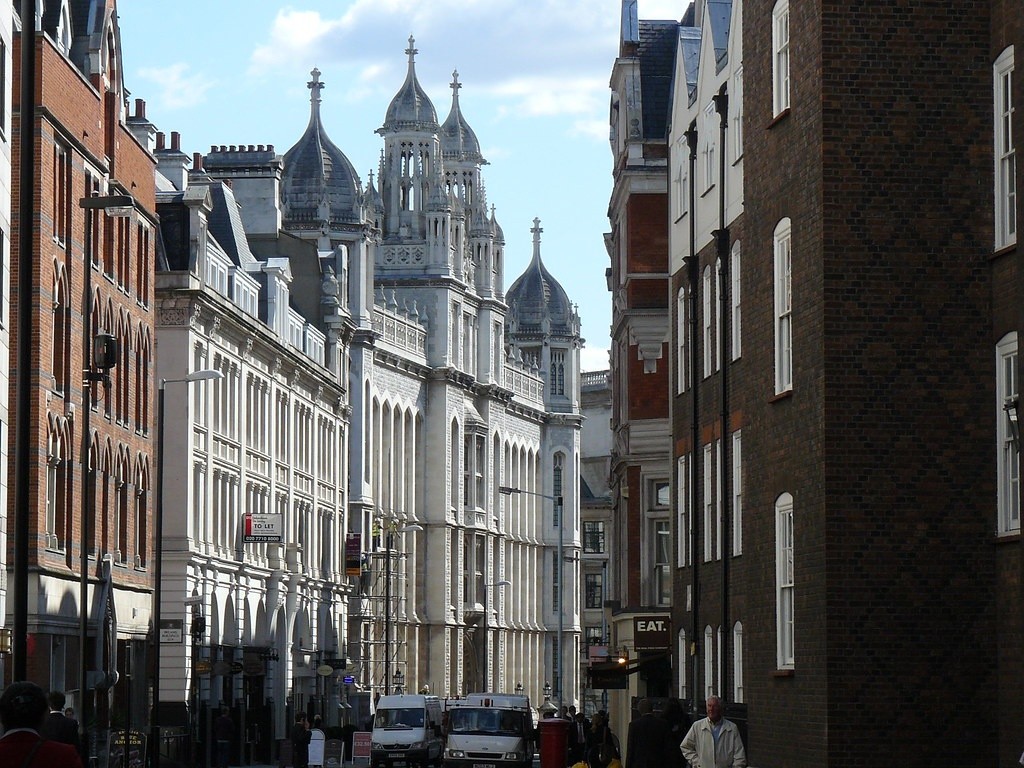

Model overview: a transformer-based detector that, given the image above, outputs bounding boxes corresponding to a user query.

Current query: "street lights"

[498,486,563,720]
[386,522,423,697]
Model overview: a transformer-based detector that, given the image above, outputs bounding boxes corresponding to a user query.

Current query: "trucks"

[440,693,538,768]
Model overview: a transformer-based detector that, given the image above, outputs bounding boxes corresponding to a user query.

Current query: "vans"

[368,694,443,768]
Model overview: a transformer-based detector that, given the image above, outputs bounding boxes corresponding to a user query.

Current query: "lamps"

[1003,393,1021,454]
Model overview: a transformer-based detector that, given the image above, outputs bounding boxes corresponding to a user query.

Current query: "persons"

[536,706,622,768]
[0,681,82,768]
[290,712,312,768]
[624,697,695,768]
[38,689,82,757]
[680,695,747,768]
[213,705,235,768]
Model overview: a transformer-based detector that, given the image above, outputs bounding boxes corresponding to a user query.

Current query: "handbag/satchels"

[598,743,610,761]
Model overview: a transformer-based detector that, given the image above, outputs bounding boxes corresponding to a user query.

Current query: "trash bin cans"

[540,718,571,768]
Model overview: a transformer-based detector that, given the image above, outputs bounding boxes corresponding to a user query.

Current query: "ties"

[573,717,575,722]
[579,724,582,744]
